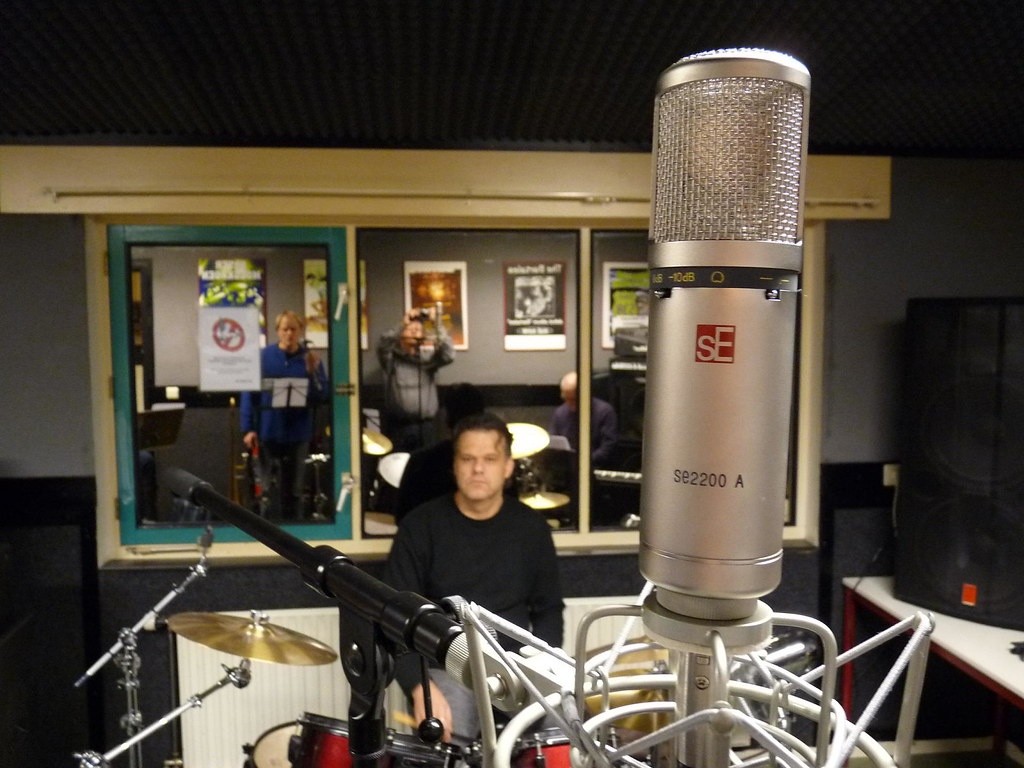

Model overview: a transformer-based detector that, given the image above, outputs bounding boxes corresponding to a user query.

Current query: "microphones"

[298,338,313,346]
[642,48,814,767]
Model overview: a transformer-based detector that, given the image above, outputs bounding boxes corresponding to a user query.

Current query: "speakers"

[893,295,1024,630]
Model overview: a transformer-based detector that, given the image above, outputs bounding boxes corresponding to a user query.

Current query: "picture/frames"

[404,260,469,351]
[602,261,650,348]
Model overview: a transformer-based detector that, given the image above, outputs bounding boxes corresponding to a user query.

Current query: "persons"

[380,411,566,751]
[376,301,646,531]
[239,310,330,523]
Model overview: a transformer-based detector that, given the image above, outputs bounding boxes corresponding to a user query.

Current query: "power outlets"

[881,464,898,485]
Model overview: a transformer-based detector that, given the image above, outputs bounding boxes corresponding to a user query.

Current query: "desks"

[842,577,1024,768]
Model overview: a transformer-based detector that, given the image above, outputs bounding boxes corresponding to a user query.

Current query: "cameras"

[415,309,430,320]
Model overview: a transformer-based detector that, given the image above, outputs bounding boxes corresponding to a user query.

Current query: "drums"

[292,709,463,768]
[469,729,570,768]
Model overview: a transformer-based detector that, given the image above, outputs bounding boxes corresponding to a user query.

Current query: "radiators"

[167,595,643,768]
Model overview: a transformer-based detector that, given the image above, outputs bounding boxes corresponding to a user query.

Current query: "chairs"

[229,396,255,509]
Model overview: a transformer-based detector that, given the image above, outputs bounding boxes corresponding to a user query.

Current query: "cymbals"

[569,638,672,733]
[378,452,412,488]
[324,424,394,456]
[506,421,552,459]
[519,491,571,510]
[164,610,339,668]
